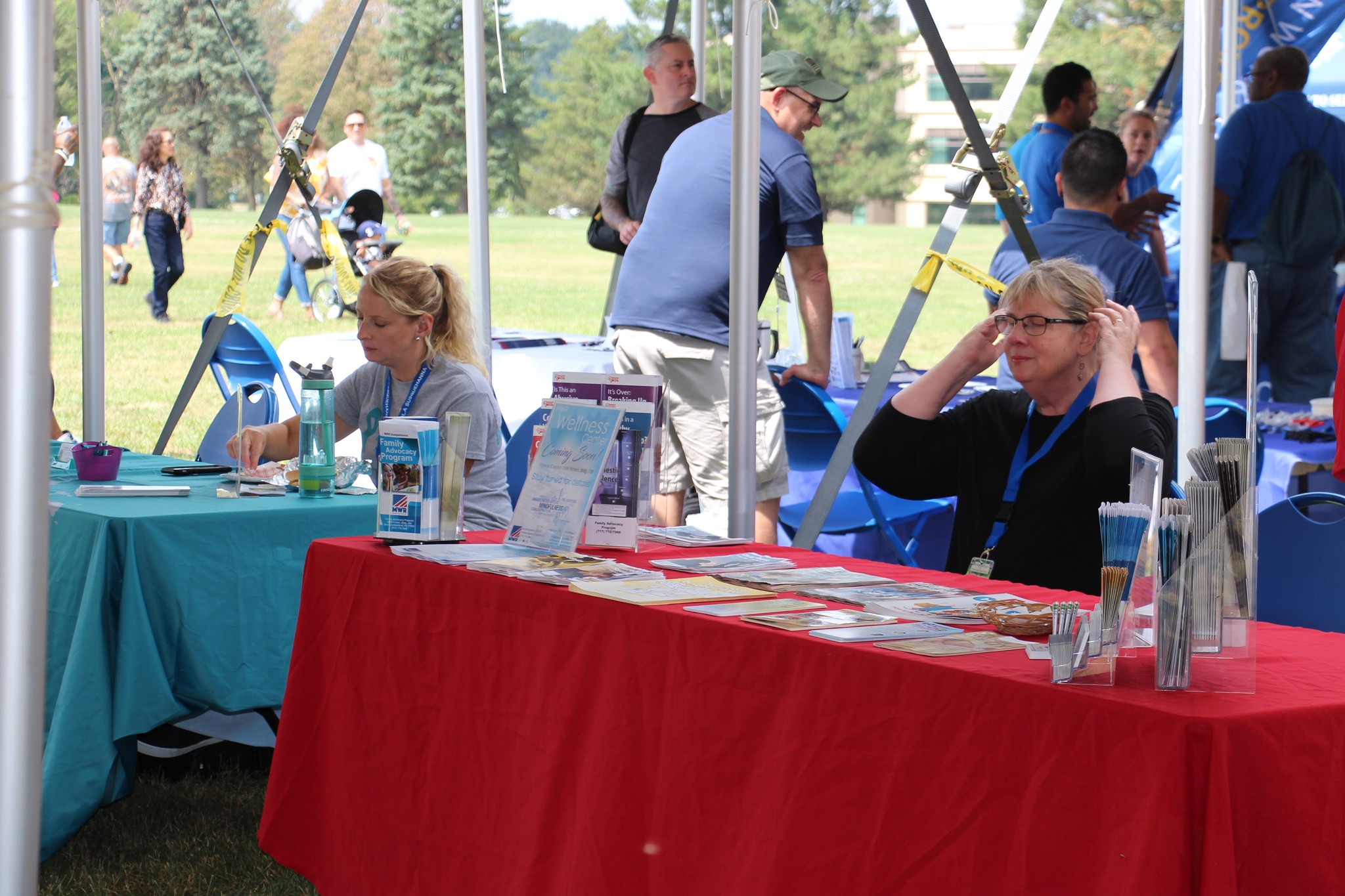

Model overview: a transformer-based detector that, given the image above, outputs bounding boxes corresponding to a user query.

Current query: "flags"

[1144,0,1345,273]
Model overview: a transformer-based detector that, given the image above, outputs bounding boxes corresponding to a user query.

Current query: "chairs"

[1256,492,1345,634]
[767,366,954,568]
[1174,397,1264,487]
[193,311,300,467]
[506,407,552,511]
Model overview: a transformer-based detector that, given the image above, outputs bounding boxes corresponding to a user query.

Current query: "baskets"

[975,598,1060,635]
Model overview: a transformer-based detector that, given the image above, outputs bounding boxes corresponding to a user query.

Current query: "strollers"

[311,189,404,324]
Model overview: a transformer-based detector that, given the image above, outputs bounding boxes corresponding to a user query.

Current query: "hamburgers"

[285,469,299,487]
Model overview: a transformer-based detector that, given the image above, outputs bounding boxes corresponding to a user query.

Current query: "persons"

[983,128,1181,407]
[137,259,512,757]
[996,62,1180,242]
[47,114,80,196]
[1112,317,1123,325]
[1117,110,1173,277]
[355,222,390,269]
[265,105,333,323]
[326,110,411,277]
[101,138,138,286]
[1215,47,1344,403]
[587,34,721,254]
[51,373,71,441]
[128,125,194,322]
[610,51,848,545]
[46,189,66,286]
[854,259,1178,596]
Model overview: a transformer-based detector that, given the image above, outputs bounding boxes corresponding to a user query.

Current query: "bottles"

[289,356,336,499]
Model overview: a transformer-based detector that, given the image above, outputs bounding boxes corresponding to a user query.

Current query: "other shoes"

[144,293,153,303]
[104,263,131,286]
[155,312,170,323]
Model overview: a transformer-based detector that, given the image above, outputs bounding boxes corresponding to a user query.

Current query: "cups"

[852,348,863,382]
[70,441,124,481]
[1309,398,1333,418]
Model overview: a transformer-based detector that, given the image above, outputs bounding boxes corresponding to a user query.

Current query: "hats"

[356,220,388,237]
[760,48,849,104]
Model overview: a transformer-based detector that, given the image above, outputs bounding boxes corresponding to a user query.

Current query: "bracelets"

[394,210,404,218]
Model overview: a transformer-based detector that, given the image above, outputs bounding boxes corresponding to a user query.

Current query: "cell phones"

[161,465,232,476]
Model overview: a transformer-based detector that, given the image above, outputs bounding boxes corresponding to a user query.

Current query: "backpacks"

[1265,99,1345,267]
[286,211,331,270]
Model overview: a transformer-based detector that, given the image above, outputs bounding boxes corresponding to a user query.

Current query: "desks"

[255,332,1345,896]
[38,439,380,858]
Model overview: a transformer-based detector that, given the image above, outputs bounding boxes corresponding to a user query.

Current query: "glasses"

[1243,71,1255,83]
[769,87,820,118]
[994,312,1087,336]
[347,123,364,129]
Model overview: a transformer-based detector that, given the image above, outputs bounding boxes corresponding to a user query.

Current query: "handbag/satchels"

[585,202,629,255]
